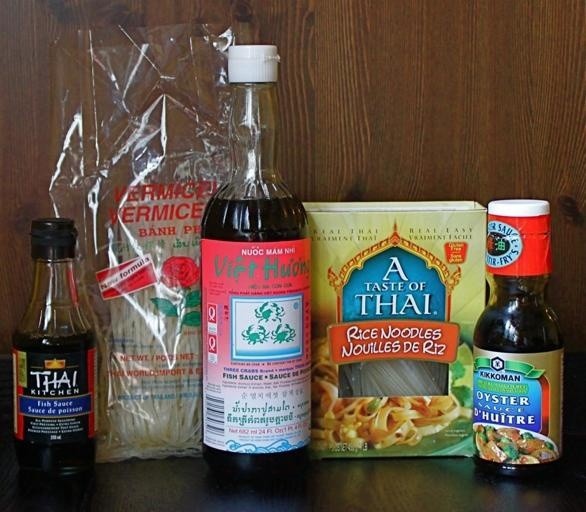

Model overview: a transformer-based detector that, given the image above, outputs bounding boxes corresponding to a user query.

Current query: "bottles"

[199,44,311,480]
[12,218,98,478]
[472,199,564,474]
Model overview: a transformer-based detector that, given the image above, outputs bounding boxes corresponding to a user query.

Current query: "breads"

[312,338,473,449]
